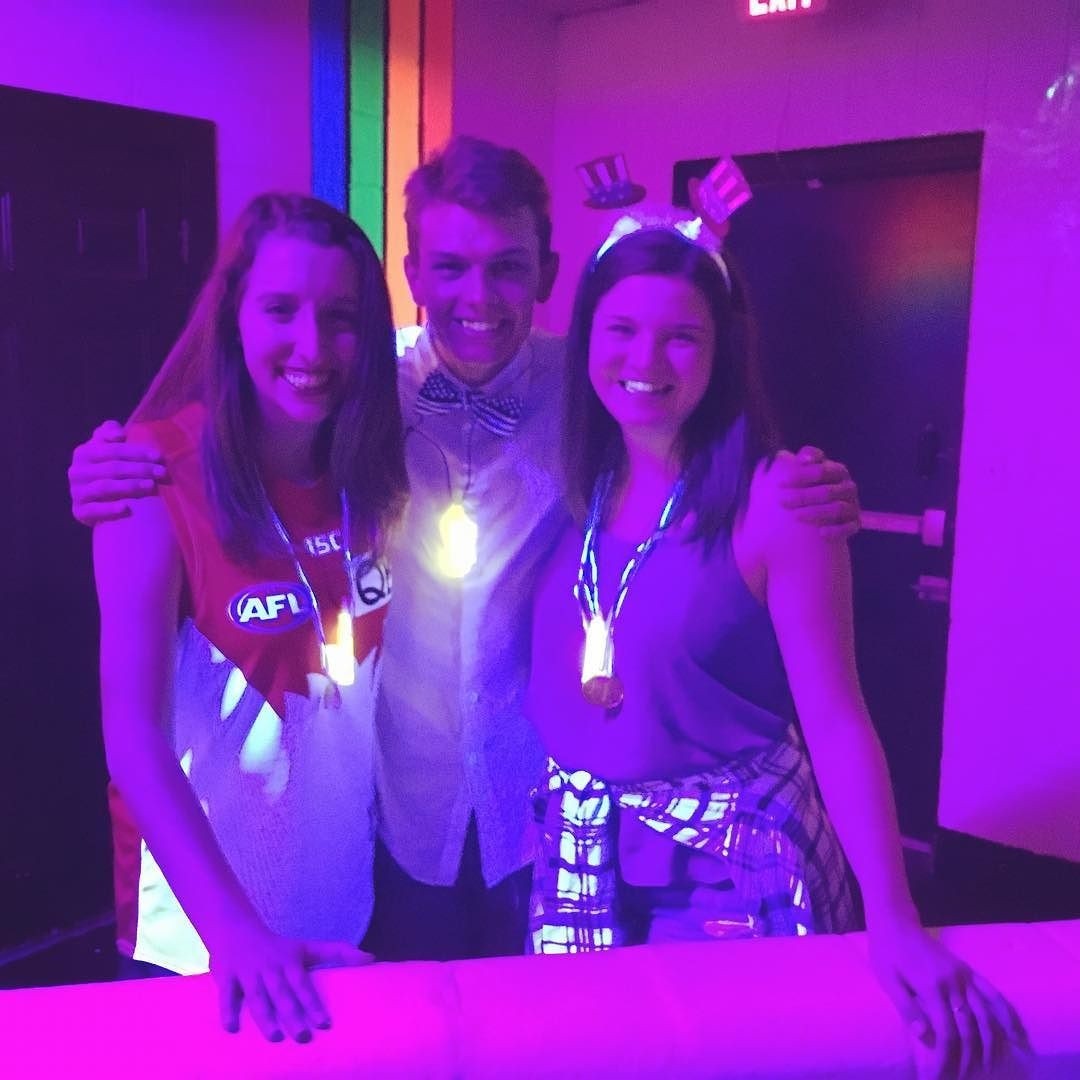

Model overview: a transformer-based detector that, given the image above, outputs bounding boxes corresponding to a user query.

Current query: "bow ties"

[412,372,526,439]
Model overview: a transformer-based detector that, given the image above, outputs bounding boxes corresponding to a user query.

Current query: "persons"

[94,191,405,1044]
[530,216,1035,1080]
[68,138,865,955]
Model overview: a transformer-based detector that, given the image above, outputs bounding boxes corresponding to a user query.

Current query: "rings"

[950,1003,967,1013]
